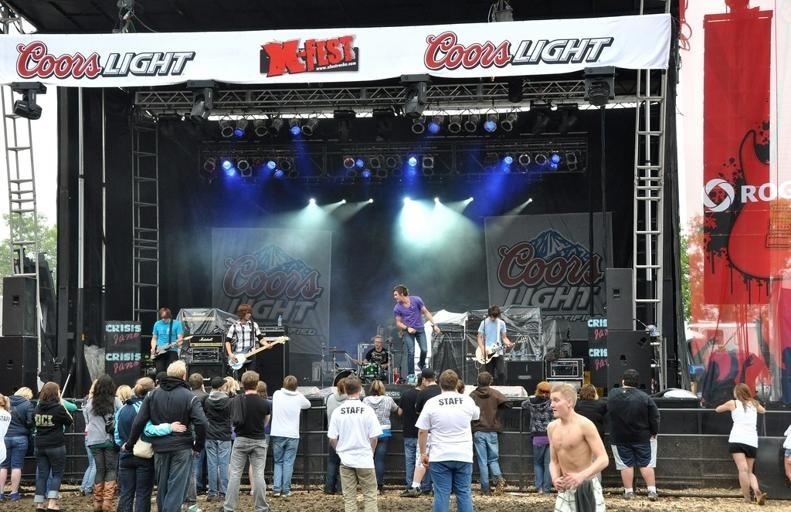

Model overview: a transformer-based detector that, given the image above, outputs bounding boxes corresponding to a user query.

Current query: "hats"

[416,368,436,379]
[211,376,227,389]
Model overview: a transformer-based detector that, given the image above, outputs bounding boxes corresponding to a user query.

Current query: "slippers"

[756,492,767,505]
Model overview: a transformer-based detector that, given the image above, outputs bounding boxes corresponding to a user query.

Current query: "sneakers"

[494,479,506,497]
[0,494,8,503]
[11,492,21,500]
[398,486,422,498]
[647,491,659,501]
[270,490,280,496]
[187,504,204,512]
[281,491,292,497]
[622,492,635,499]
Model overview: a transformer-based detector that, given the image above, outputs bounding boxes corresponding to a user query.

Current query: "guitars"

[475,337,529,364]
[726,127,790,283]
[228,336,290,370]
[150,335,194,360]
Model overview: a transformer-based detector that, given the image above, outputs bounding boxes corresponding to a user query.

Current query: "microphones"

[483,314,486,320]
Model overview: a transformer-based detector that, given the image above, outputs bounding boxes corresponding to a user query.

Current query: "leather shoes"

[47,506,65,512]
[36,507,46,512]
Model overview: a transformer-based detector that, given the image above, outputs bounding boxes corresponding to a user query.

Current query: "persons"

[782,423,791,485]
[456,379,464,394]
[324,378,346,495]
[115,384,132,405]
[360,379,403,496]
[219,370,272,512]
[574,383,608,483]
[203,375,232,502]
[224,377,239,445]
[0,386,34,502]
[116,371,167,402]
[607,368,660,502]
[327,373,383,512]
[0,393,12,467]
[80,378,99,497]
[469,372,513,497]
[271,375,312,497]
[352,335,393,383]
[114,375,187,512]
[249,381,273,495]
[392,285,441,378]
[86,375,124,512]
[714,383,767,504]
[414,369,481,511]
[521,381,553,495]
[397,368,441,498]
[545,382,608,512]
[394,375,428,497]
[33,381,78,512]
[187,372,210,496]
[150,307,184,373]
[477,305,516,386]
[120,359,210,512]
[225,304,273,380]
[398,374,425,490]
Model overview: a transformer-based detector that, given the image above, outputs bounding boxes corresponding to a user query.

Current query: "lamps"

[428,116,443,134]
[360,170,371,181]
[407,154,419,167]
[375,169,389,180]
[501,114,519,131]
[483,114,499,133]
[288,117,300,136]
[367,157,381,169]
[584,68,616,106]
[518,153,531,166]
[530,103,554,135]
[401,74,432,118]
[186,79,216,127]
[423,169,435,177]
[391,168,402,179]
[505,156,512,165]
[301,119,320,137]
[421,156,435,168]
[564,152,578,166]
[557,102,583,136]
[280,157,295,171]
[551,153,562,165]
[286,169,300,180]
[268,118,283,136]
[568,164,578,171]
[205,156,217,164]
[447,115,462,133]
[372,110,394,118]
[219,119,234,139]
[233,116,249,138]
[534,154,547,166]
[203,160,216,173]
[463,115,480,134]
[382,156,397,168]
[356,159,365,169]
[236,158,250,170]
[251,118,268,137]
[240,166,253,177]
[411,115,427,134]
[9,81,46,120]
[344,158,355,169]
[345,168,357,179]
[334,109,356,120]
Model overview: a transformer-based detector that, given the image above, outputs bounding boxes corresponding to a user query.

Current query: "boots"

[102,480,117,512]
[92,480,103,512]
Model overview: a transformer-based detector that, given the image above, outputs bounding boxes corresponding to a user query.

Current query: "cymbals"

[329,350,346,354]
[387,349,401,353]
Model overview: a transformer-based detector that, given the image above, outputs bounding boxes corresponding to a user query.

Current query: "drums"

[360,364,381,378]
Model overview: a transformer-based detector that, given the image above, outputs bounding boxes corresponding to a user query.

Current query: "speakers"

[1,337,38,399]
[607,330,650,394]
[3,277,37,336]
[606,268,632,329]
